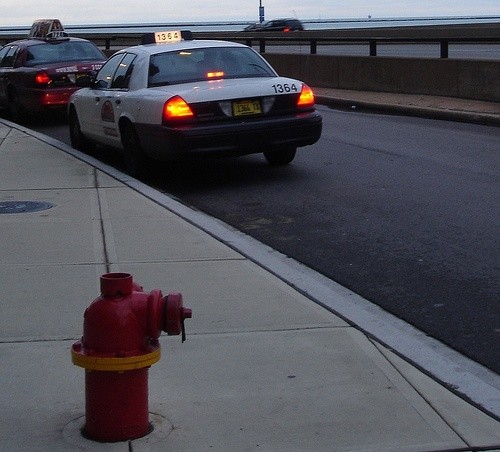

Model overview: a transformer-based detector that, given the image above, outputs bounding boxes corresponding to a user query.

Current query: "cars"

[0,31,109,126]
[67,30,322,182]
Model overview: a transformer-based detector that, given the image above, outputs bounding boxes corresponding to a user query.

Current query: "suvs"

[244,18,304,33]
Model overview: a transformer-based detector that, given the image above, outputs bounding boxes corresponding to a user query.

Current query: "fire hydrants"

[71,272,192,442]
